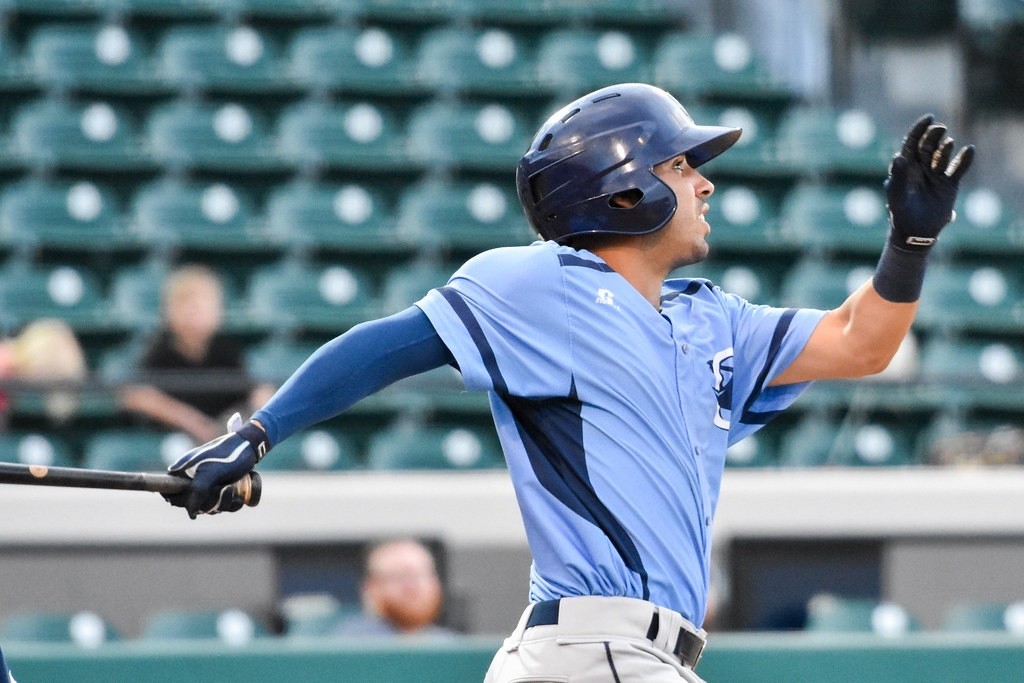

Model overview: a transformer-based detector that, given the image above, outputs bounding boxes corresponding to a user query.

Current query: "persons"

[99,261,277,471]
[157,83,979,683]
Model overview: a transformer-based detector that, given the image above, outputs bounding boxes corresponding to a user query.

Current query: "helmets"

[516,82,742,244]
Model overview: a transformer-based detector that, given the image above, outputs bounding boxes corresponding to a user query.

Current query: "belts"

[525,597,707,671]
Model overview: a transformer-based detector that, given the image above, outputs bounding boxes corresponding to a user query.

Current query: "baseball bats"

[0,456,262,508]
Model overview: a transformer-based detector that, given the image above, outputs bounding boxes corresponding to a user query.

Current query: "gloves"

[160,412,271,520]
[871,115,975,303]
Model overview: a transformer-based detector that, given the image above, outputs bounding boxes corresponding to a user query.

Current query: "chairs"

[0,0,1024,646]
